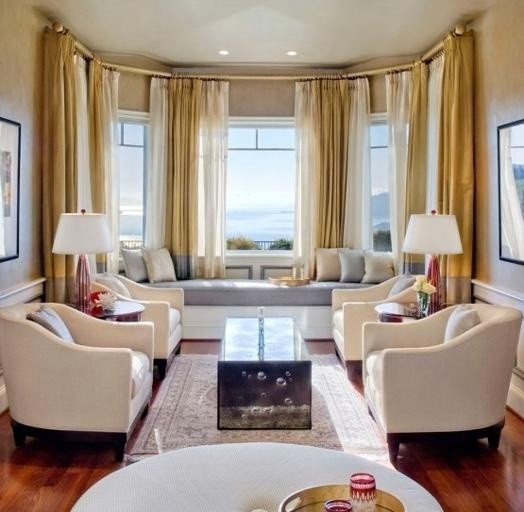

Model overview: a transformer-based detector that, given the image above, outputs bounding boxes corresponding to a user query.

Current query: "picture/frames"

[495,118,523,268]
[0,116,22,263]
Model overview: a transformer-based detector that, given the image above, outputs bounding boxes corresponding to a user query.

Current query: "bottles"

[349,473,377,512]
[322,498,353,511]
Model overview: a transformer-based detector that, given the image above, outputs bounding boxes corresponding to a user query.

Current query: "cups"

[292,264,303,280]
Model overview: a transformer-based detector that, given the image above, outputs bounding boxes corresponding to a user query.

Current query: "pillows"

[25,304,77,345]
[445,307,482,344]
[314,247,394,284]
[99,276,132,298]
[116,246,179,284]
[381,273,415,298]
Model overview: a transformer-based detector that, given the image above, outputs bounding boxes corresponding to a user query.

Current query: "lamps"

[47,208,111,304]
[397,207,465,312]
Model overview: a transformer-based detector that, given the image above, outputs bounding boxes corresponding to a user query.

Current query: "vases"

[420,296,427,314]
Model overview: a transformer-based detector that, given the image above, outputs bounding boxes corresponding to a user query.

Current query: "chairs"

[360,300,524,466]
[96,271,185,378]
[328,273,423,382]
[1,301,158,463]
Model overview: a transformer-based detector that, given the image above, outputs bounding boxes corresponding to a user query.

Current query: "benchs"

[119,272,380,343]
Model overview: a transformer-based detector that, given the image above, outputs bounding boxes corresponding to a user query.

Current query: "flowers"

[413,279,439,311]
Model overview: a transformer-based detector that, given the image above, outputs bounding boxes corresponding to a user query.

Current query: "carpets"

[121,352,391,462]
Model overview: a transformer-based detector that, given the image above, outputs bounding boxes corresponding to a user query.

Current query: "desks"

[376,298,419,322]
[86,297,146,323]
[64,440,446,512]
